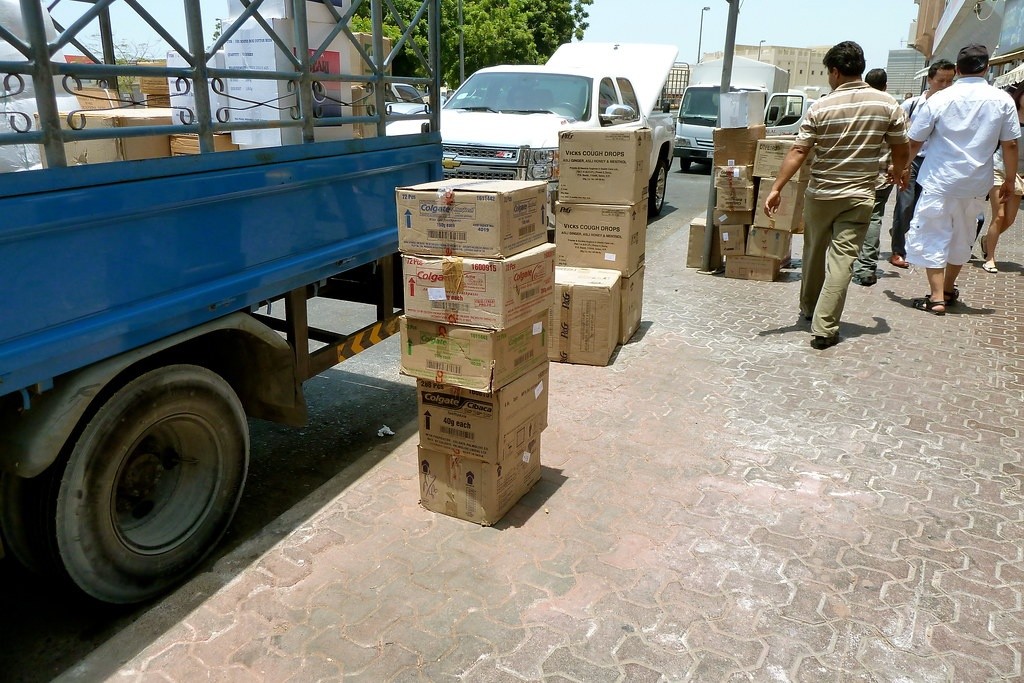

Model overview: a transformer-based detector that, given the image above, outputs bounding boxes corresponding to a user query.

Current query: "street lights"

[758,40,766,60]
[697,6,711,63]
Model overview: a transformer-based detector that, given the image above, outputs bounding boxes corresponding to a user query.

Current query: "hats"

[957,42,989,71]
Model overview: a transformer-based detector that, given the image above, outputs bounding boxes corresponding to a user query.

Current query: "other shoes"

[815,332,839,348]
[861,277,877,286]
[983,263,998,273]
[981,235,988,262]
[805,316,812,321]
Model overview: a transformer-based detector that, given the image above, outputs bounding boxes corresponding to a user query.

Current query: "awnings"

[996,63,1024,87]
[914,66,931,80]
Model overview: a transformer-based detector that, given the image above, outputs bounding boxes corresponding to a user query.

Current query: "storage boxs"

[33,1,391,167]
[549,127,652,366]
[396,179,555,525]
[686,89,817,283]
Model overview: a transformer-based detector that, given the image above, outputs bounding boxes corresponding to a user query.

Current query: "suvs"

[385,42,682,232]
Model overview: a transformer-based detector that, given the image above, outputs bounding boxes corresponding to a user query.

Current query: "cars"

[385,83,427,113]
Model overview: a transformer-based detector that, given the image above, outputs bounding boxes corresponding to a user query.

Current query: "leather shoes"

[891,254,909,268]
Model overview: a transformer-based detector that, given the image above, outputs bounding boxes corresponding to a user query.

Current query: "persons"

[905,43,1021,316]
[854,68,894,287]
[764,41,910,349]
[905,93,912,100]
[889,60,956,267]
[981,82,1024,273]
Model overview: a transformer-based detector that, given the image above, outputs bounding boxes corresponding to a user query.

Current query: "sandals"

[944,288,959,306]
[912,295,946,316]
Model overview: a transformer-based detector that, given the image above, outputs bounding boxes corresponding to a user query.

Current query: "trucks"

[673,55,807,173]
[0,0,447,611]
